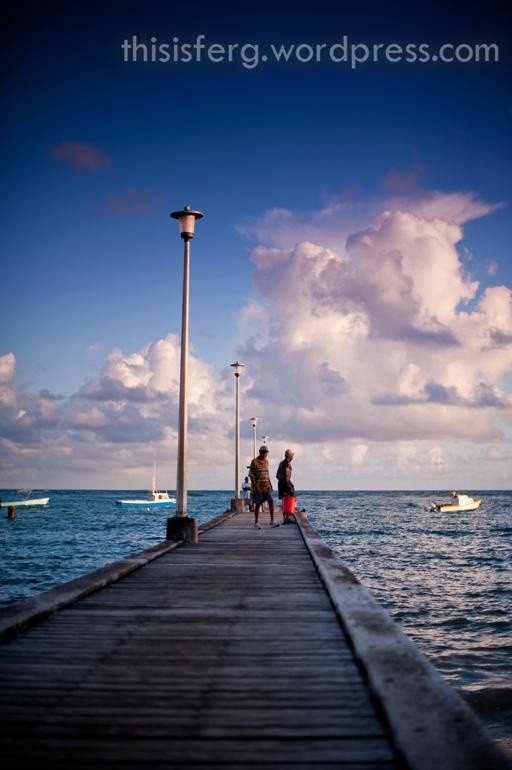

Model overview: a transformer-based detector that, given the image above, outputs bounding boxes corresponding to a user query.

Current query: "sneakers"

[270,522,280,528]
[254,523,262,530]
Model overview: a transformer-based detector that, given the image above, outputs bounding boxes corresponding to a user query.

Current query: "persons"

[276,449,295,525]
[248,501,265,512]
[247,446,279,529]
[242,477,251,504]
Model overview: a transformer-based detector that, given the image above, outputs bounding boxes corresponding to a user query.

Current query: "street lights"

[260,434,268,444]
[249,415,258,458]
[230,359,246,513]
[165,203,208,543]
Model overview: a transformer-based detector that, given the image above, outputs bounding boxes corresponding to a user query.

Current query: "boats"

[427,489,483,513]
[115,460,177,507]
[0,497,51,507]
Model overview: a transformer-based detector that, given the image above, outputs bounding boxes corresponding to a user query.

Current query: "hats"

[259,447,269,452]
